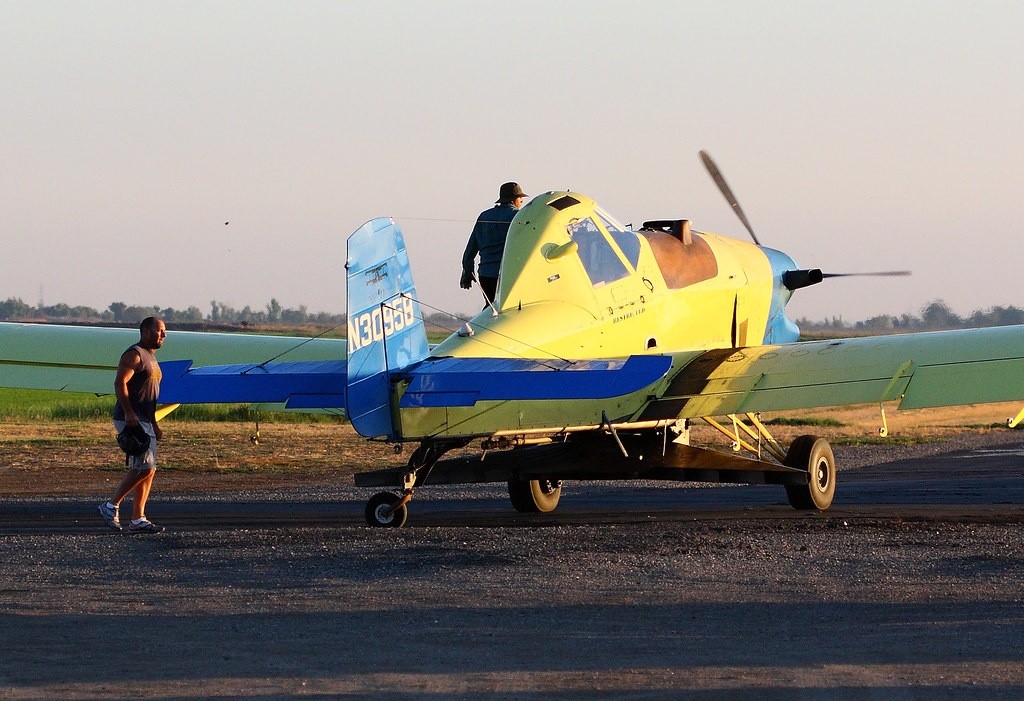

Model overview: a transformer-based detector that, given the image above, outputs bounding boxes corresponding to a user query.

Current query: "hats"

[495,182,528,204]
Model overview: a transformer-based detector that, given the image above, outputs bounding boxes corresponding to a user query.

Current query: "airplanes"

[0,148,1024,529]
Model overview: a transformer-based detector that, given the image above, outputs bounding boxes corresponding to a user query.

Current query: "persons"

[460,182,528,311]
[99,317,165,534]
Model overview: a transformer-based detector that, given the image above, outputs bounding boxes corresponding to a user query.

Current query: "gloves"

[460,269,477,290]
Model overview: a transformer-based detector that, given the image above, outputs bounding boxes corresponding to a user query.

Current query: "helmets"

[116,421,151,457]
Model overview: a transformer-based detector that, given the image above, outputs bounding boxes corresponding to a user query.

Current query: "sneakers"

[98,500,122,532]
[129,517,165,533]
